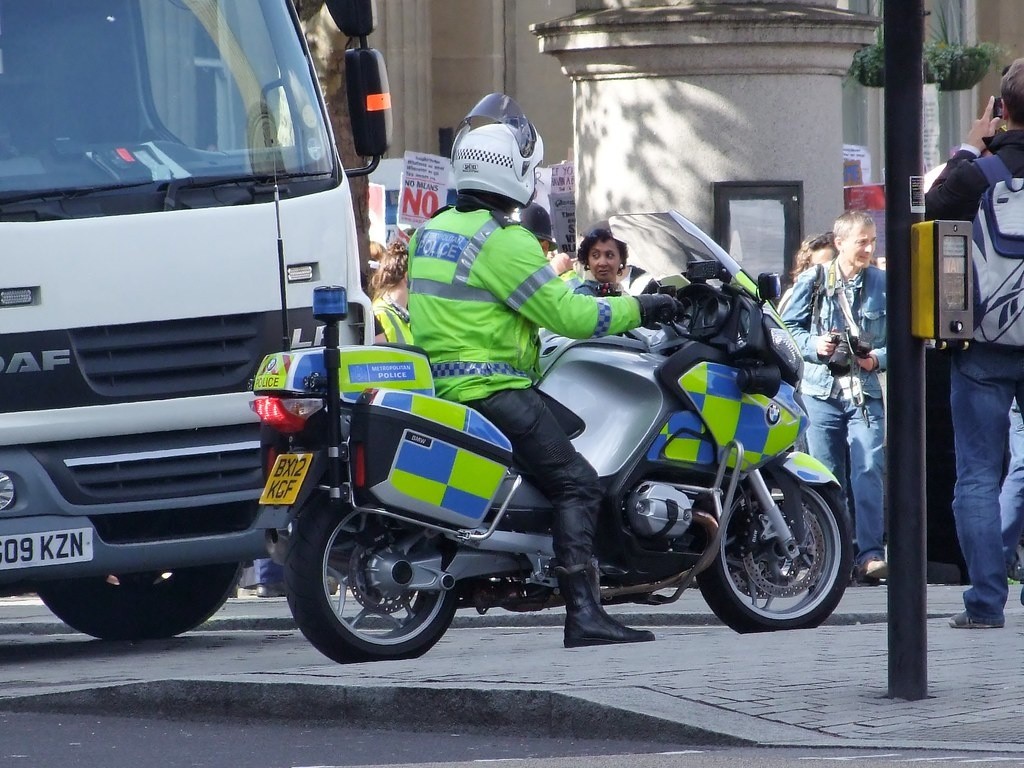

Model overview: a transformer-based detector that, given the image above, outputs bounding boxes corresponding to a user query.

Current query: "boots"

[553,559,656,648]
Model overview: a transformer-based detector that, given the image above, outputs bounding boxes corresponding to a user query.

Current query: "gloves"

[635,293,679,330]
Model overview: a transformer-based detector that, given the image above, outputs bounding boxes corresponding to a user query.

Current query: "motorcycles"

[247,185,853,663]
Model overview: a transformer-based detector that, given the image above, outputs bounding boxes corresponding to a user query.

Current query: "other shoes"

[949,609,1004,628]
[257,583,285,596]
[326,576,338,596]
[853,556,887,582]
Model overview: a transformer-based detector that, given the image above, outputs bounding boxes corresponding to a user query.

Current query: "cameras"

[827,334,872,376]
[993,98,1002,118]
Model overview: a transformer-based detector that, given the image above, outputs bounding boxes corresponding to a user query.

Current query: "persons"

[374,231,410,348]
[254,526,346,601]
[1,18,145,162]
[507,199,639,336]
[923,60,1024,629]
[408,94,682,649]
[778,203,888,585]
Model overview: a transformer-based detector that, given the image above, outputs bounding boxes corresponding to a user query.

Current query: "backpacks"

[974,154,1024,348]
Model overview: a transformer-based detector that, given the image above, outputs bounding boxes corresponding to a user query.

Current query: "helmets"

[510,202,558,250]
[450,117,543,206]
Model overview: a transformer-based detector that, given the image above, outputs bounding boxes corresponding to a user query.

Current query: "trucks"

[0,0,392,640]
